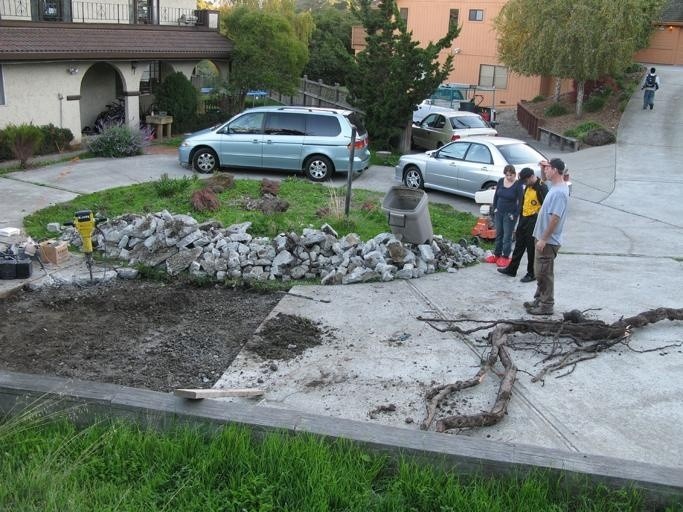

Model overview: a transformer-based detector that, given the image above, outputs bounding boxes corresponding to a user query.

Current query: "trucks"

[418,85,500,130]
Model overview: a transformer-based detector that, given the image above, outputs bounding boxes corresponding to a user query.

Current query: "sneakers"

[485,255,516,277]
[524,298,553,315]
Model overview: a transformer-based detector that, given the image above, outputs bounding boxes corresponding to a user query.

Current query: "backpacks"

[646,74,657,87]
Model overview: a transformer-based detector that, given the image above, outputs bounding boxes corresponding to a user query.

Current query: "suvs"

[176,102,373,184]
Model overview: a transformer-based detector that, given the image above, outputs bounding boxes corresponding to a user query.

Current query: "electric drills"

[64,210,107,285]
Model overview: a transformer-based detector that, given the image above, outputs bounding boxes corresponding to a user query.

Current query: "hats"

[541,158,565,170]
[518,168,534,182]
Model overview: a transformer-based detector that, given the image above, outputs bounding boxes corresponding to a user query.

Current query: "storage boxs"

[40,239,69,264]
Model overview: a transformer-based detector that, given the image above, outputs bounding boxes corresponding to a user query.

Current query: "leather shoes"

[520,275,537,282]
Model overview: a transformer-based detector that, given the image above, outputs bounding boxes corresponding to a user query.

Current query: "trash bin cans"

[380,186,433,245]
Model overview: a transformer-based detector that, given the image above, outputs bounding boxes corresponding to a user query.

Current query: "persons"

[641,68,660,110]
[486,158,569,315]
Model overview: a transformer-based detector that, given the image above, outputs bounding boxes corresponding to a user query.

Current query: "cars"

[391,132,575,210]
[409,107,501,151]
